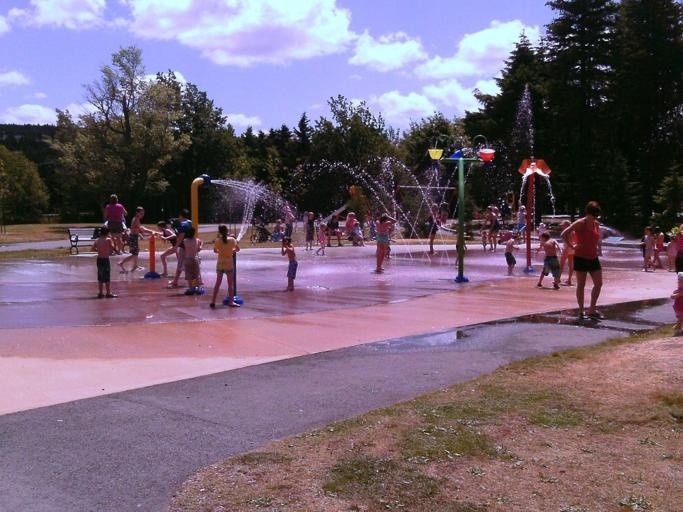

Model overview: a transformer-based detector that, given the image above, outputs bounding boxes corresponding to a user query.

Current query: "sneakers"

[591,314,604,319]
[579,313,588,319]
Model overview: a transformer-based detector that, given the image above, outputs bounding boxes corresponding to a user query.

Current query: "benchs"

[316,227,348,246]
[68,228,101,254]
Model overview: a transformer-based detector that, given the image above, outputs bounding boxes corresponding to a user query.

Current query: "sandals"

[229,303,240,307]
[210,304,215,307]
[106,293,117,297]
[98,293,104,296]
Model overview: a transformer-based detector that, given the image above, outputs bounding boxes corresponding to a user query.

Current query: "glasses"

[591,213,599,217]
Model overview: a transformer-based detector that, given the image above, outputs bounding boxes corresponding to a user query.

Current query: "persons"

[538,223,545,240]
[560,201,605,319]
[482,231,488,251]
[352,221,366,247]
[428,203,439,254]
[515,206,527,234]
[552,219,575,285]
[158,221,180,277]
[675,224,683,273]
[92,227,118,298]
[303,212,314,251]
[639,226,656,272]
[652,226,664,267]
[277,219,285,236]
[117,206,143,272]
[667,235,677,271]
[327,214,342,246]
[183,228,203,295]
[281,236,298,292]
[316,223,326,256]
[168,208,193,285]
[536,232,562,289]
[285,216,291,236]
[455,236,467,266]
[271,227,278,242]
[385,246,391,259]
[103,194,127,254]
[375,213,391,272]
[480,206,500,253]
[505,232,520,275]
[210,224,240,309]
[671,273,683,330]
[104,219,129,254]
[346,212,359,245]
